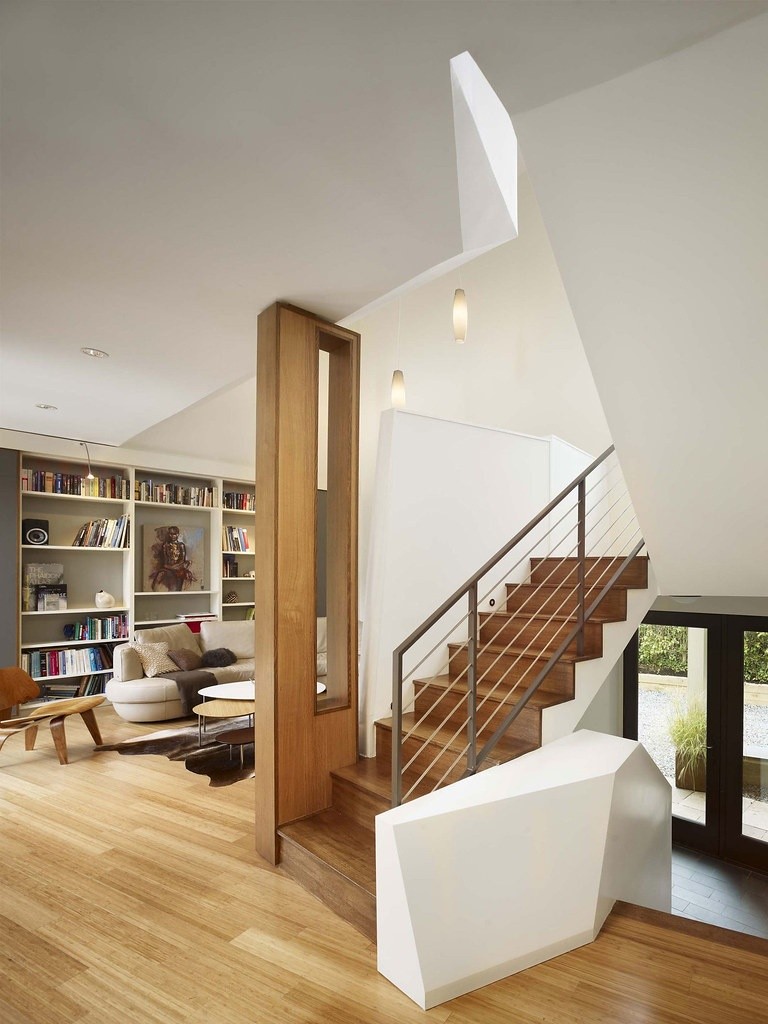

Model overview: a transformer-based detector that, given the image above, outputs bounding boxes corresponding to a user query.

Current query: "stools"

[216,726,255,768]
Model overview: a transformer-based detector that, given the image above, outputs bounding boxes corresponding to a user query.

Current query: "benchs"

[0,665,105,765]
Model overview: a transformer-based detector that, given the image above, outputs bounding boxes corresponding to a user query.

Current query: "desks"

[191,681,326,747]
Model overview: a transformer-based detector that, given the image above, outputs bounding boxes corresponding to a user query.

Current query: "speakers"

[21,519,49,545]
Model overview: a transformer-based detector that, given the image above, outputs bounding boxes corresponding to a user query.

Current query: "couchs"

[105,617,330,722]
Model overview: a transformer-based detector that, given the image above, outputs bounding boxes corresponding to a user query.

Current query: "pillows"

[201,648,237,667]
[128,641,181,678]
[134,623,202,657]
[167,648,201,671]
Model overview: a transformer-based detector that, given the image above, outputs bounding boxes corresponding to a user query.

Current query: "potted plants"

[669,697,768,803]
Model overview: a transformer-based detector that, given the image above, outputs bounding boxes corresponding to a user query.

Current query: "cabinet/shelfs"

[15,451,255,717]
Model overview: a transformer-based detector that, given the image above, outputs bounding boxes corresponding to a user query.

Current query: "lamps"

[390,299,407,408]
[452,267,467,341]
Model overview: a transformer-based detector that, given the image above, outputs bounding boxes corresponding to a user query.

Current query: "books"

[223,553,239,578]
[21,469,131,500]
[72,514,130,549]
[74,613,128,640]
[223,491,256,511]
[22,563,63,611]
[135,480,217,508]
[222,525,250,553]
[42,673,113,698]
[176,612,216,620]
[21,643,120,678]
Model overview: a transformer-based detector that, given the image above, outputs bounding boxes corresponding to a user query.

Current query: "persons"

[162,526,190,570]
